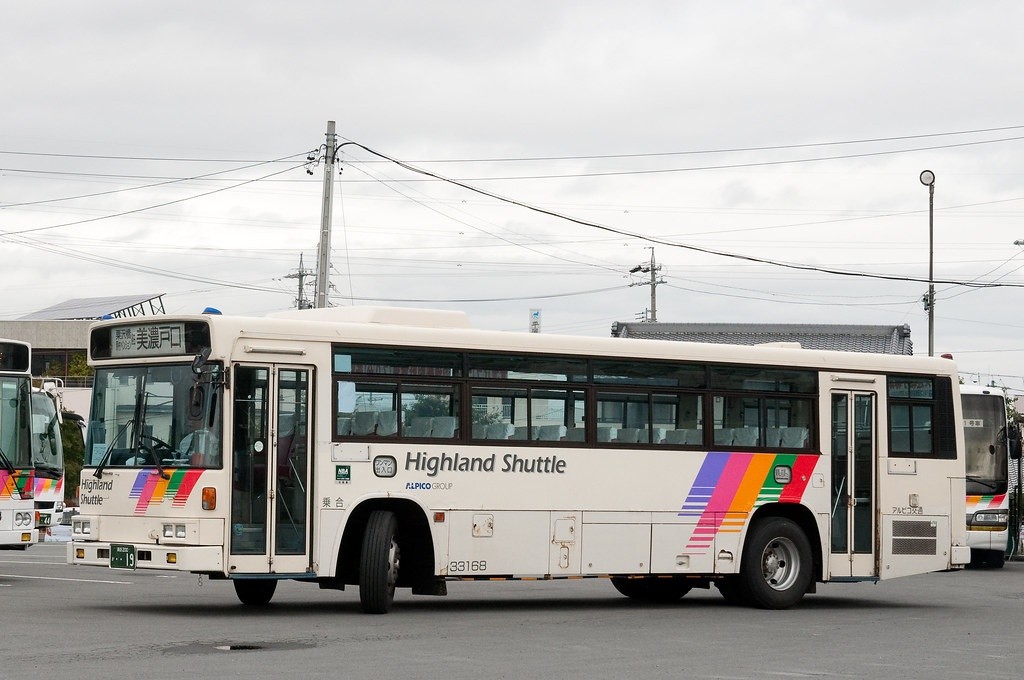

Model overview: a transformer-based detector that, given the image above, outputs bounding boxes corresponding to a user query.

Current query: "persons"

[177,404,219,467]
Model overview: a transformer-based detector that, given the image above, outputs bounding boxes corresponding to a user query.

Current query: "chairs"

[339,411,810,449]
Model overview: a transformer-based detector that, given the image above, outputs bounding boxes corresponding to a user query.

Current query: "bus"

[952,376,1024,568]
[0,336,42,552]
[67,308,973,610]
[26,375,66,531]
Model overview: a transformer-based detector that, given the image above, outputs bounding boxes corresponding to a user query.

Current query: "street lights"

[919,169,937,359]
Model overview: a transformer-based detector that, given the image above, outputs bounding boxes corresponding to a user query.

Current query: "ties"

[186,436,196,455]
